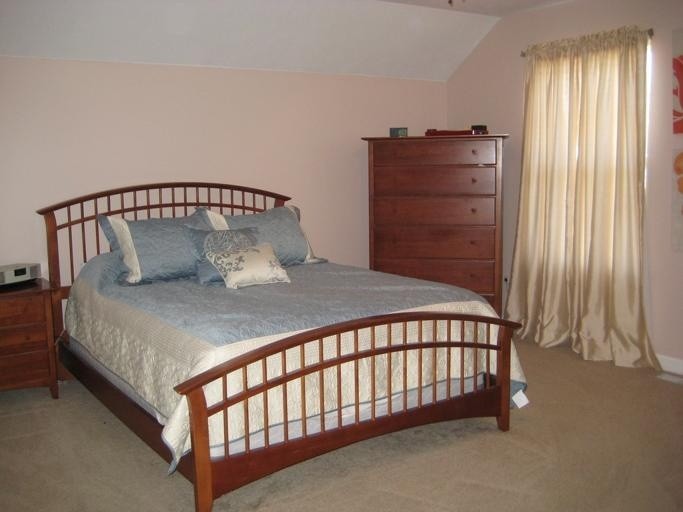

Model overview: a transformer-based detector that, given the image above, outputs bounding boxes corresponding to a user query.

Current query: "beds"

[37,182,526,512]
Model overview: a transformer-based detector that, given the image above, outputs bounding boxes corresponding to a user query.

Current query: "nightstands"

[0,278,59,399]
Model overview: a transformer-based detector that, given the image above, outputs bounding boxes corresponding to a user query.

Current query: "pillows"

[96,207,328,291]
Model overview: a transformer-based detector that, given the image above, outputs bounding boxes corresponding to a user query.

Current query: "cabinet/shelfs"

[362,134,509,318]
